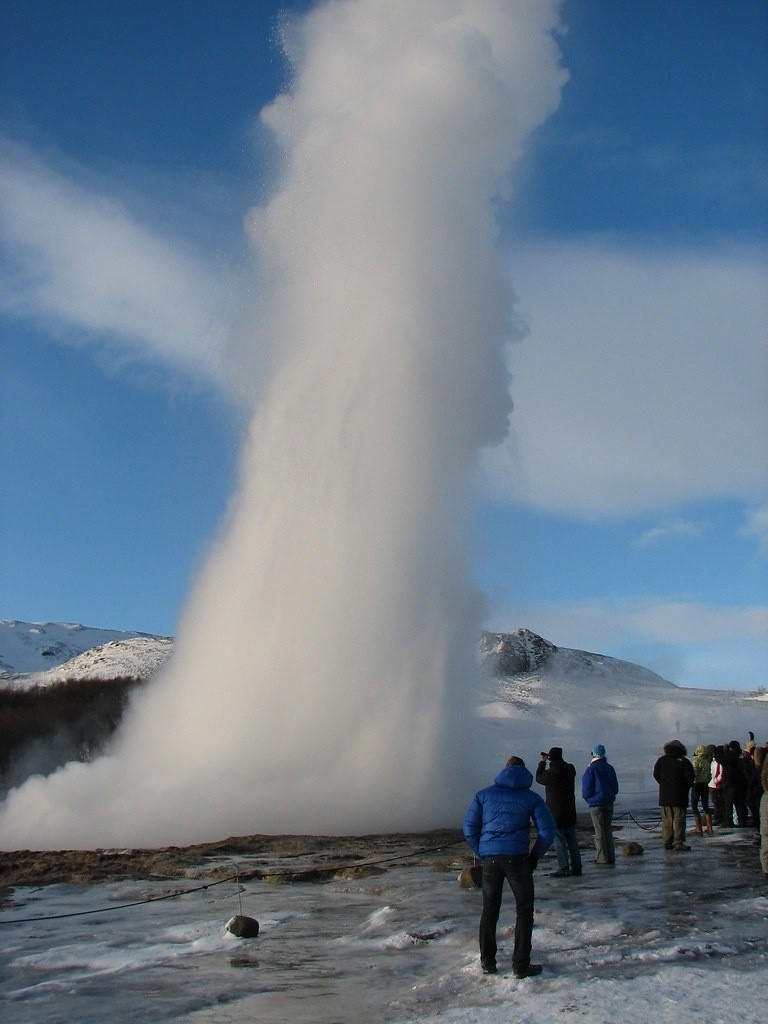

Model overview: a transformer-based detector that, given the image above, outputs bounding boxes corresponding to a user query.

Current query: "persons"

[582,744,618,864]
[463,756,556,979]
[759,753,768,877]
[536,747,583,876]
[653,740,695,850]
[676,720,680,732]
[691,745,713,833]
[696,727,701,741]
[708,740,768,828]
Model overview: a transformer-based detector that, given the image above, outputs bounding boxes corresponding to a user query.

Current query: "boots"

[689,814,702,836]
[705,814,712,835]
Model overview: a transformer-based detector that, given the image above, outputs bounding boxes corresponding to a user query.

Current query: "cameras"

[540,752,549,758]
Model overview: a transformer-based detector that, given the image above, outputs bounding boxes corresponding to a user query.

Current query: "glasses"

[591,752,593,755]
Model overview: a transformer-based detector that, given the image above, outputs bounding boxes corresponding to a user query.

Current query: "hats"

[594,744,605,756]
[549,748,562,761]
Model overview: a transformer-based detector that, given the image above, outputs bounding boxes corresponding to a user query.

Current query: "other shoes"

[483,964,497,972]
[514,964,543,979]
[675,843,691,851]
[550,869,569,876]
[664,843,675,849]
[570,868,582,876]
